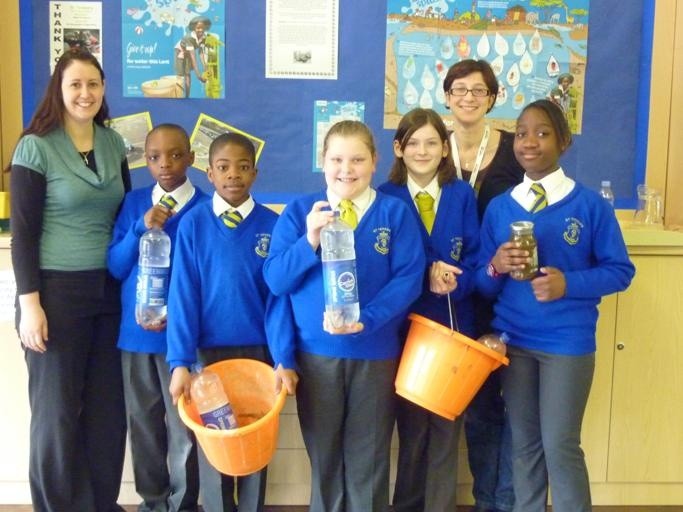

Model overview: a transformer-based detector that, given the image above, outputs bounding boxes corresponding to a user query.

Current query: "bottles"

[320,211,358,329]
[135,224,171,326]
[186,361,239,431]
[631,185,664,235]
[473,331,510,357]
[597,181,615,209]
[508,221,539,284]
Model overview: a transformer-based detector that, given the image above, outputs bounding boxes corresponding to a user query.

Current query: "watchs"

[484,260,502,281]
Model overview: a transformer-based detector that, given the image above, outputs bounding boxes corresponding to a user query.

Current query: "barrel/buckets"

[394,274,509,421]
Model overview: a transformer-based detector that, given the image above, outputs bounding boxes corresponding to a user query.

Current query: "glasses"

[446,87,494,98]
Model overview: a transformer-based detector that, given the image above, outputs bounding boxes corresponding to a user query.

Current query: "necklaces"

[454,136,480,169]
[71,138,93,165]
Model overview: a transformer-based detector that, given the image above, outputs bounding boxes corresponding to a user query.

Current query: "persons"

[478,97,635,511]
[104,123,213,511]
[169,15,210,97]
[437,57,525,223]
[6,48,131,512]
[373,106,479,512]
[546,71,574,115]
[261,118,427,511]
[162,131,303,511]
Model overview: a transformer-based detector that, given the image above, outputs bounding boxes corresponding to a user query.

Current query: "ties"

[335,198,359,231]
[526,183,549,214]
[220,210,242,228]
[158,195,177,209]
[413,190,436,236]
